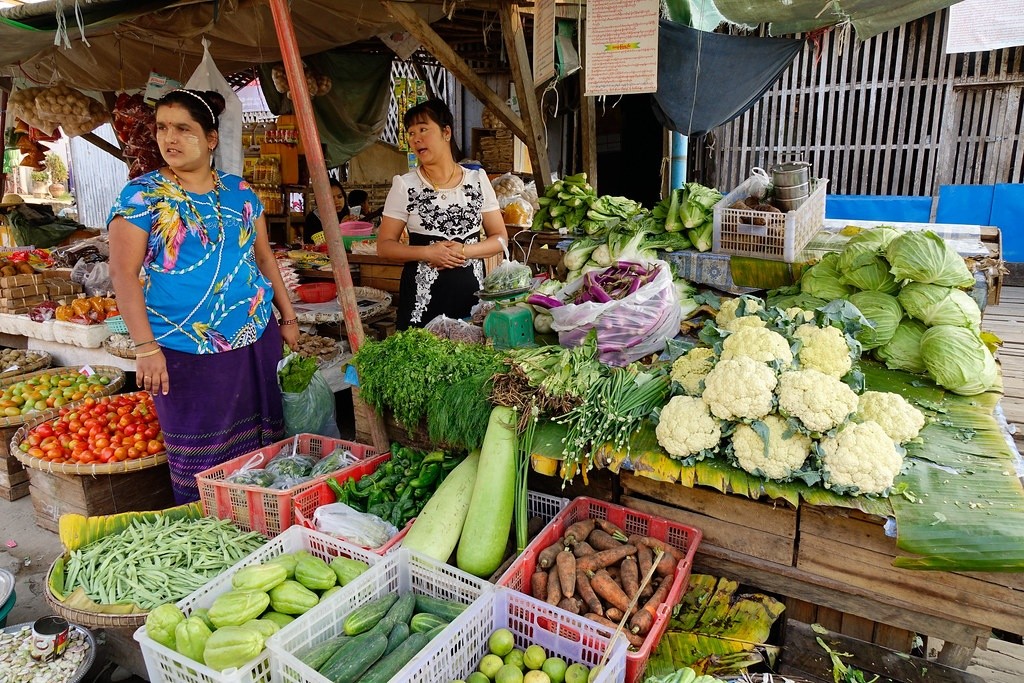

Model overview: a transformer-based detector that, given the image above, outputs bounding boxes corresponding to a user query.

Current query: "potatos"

[0,348,42,373]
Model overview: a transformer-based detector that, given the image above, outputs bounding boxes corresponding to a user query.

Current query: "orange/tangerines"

[0,367,110,416]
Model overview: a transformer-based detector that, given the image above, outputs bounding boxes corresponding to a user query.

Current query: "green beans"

[62,513,270,611]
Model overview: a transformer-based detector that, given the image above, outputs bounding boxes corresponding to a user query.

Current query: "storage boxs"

[710,175,830,264]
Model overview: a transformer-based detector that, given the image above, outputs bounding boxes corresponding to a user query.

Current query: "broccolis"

[654,293,923,496]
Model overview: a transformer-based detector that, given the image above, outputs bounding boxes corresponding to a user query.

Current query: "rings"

[143,375,151,379]
[297,339,299,342]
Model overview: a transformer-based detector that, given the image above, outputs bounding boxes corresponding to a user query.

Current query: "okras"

[531,172,596,232]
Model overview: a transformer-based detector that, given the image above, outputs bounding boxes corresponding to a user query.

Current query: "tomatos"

[18,390,167,466]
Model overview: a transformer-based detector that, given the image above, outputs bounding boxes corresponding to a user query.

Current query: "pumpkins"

[145,548,368,683]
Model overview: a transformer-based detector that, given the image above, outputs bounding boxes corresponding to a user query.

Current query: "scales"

[474,288,534,348]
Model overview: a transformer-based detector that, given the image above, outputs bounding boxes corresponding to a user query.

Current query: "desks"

[342,310,1024,683]
[540,208,1003,312]
[0,314,353,396]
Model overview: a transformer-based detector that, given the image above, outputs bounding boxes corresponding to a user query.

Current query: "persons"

[377,96,508,334]
[107,88,305,505]
[348,190,373,222]
[302,177,351,244]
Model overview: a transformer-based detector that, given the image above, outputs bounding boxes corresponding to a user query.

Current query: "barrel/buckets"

[770,160,812,213]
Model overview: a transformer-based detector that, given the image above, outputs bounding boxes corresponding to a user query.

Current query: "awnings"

[0,2,555,454]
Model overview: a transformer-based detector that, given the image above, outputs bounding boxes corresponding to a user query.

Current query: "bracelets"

[136,347,161,357]
[462,243,465,256]
[134,339,156,347]
[278,316,299,324]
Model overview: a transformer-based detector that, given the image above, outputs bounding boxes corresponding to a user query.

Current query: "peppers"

[327,440,466,530]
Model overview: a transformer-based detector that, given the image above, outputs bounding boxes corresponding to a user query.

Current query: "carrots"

[530,517,685,651]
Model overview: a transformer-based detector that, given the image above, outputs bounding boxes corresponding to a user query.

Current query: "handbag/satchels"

[424,313,452,339]
[277,350,342,441]
[481,236,532,292]
[449,319,483,345]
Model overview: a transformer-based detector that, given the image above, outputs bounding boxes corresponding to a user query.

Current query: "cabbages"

[800,226,998,396]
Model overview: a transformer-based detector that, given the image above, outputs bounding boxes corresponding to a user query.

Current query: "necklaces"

[423,163,456,193]
[169,166,223,244]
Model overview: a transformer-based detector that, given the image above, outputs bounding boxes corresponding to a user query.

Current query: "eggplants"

[520,260,680,365]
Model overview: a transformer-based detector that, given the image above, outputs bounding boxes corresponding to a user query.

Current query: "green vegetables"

[278,355,333,437]
[349,325,672,486]
[564,181,725,285]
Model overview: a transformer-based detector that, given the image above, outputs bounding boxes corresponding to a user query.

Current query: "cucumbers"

[290,589,470,683]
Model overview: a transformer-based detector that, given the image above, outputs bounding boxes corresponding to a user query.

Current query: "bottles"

[242,121,296,215]
